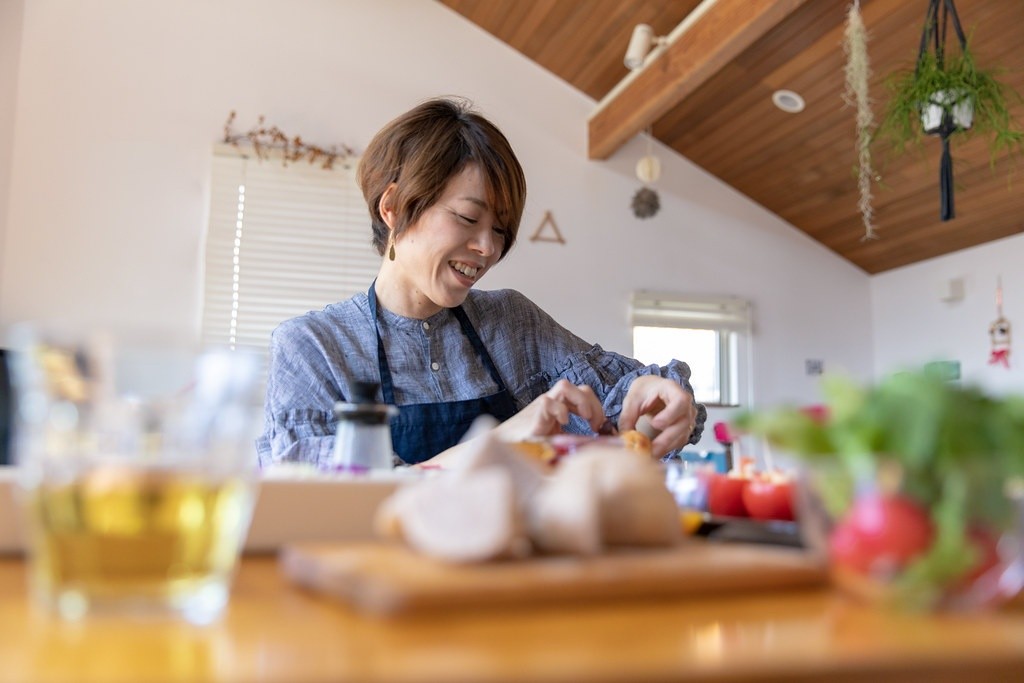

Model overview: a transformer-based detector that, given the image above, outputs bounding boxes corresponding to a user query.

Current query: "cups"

[17,346,271,633]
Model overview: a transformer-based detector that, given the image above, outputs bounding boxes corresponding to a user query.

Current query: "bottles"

[330,380,398,473]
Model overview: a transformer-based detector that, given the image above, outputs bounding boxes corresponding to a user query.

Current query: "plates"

[1,467,398,556]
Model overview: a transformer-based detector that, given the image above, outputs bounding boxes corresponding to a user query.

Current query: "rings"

[689,426,692,431]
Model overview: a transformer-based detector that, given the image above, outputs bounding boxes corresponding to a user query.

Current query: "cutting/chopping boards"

[279,537,828,620]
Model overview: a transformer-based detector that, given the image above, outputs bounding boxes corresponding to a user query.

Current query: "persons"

[259,97,707,471]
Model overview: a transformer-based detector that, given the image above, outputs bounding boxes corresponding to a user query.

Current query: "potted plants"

[851,3,1024,202]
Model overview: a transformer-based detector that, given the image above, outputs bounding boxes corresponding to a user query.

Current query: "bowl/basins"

[763,441,1024,612]
